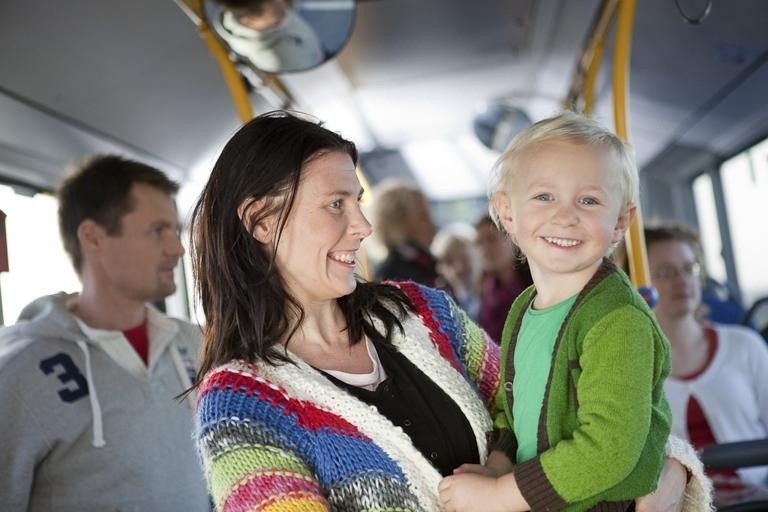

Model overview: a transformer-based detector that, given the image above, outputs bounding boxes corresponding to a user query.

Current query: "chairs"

[701,277,768,338]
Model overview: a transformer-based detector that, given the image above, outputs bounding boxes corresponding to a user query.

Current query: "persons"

[368,185,531,343]
[170,107,718,511]
[437,106,673,511]
[0,151,213,512]
[614,220,767,506]
